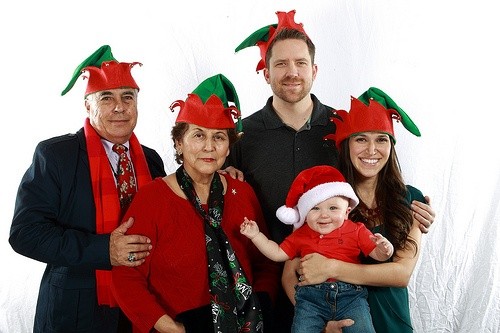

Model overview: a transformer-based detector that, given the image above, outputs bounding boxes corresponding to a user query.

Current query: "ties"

[112,144,137,206]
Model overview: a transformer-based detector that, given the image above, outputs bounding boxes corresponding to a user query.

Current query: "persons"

[240,164,394,333]
[221,9,436,333]
[9,45,245,333]
[281,87,429,333]
[112,74,280,333]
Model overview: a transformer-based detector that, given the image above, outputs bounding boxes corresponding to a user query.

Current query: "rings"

[128,253,135,262]
[299,276,303,282]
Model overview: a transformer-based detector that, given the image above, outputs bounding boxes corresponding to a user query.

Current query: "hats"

[169,73,244,138]
[276,165,360,232]
[323,87,422,156]
[60,44,143,99]
[235,9,313,74]
